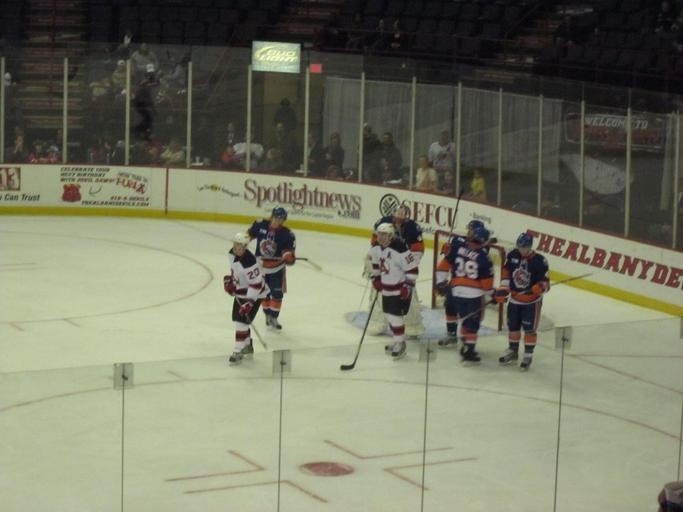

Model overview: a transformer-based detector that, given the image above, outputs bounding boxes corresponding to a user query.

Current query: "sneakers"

[438,333,457,345]
[385,341,405,356]
[499,350,518,362]
[266,314,282,329]
[460,343,481,360]
[229,339,254,362]
[521,354,532,368]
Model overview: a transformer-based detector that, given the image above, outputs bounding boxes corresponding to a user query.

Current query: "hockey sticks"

[447,186,466,242]
[233,291,276,354]
[503,272,592,300]
[455,298,496,338]
[341,291,381,370]
[255,257,322,271]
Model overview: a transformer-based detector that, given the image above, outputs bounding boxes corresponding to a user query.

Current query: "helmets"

[393,205,405,223]
[468,220,488,243]
[271,208,287,220]
[516,233,532,247]
[234,233,250,246]
[378,223,394,236]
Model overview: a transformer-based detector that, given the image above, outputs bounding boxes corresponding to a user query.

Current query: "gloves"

[239,301,254,317]
[532,283,543,296]
[373,277,381,290]
[224,276,236,295]
[494,285,509,303]
[400,283,411,301]
[437,281,450,295]
[281,250,294,265]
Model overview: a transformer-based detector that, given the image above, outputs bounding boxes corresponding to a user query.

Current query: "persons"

[223,230,272,363]
[434,219,490,348]
[363,222,420,358]
[493,232,553,373]
[371,205,425,264]
[246,206,297,329]
[449,228,493,363]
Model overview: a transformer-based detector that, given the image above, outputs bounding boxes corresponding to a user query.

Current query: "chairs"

[0,0,683,252]
